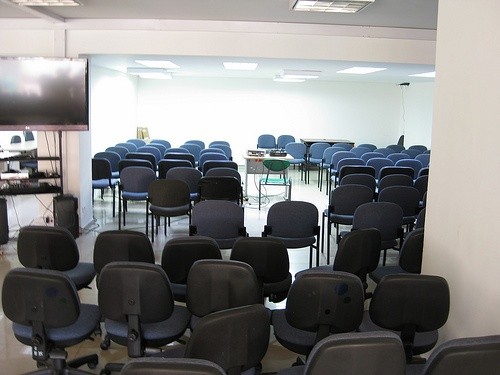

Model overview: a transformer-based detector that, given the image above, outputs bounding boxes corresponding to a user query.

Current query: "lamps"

[290,0,374,15]
[125,67,173,81]
[273,69,322,84]
[7,0,85,9]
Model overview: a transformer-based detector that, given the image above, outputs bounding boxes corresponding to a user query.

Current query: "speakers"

[0,198,9,245]
[53,195,80,239]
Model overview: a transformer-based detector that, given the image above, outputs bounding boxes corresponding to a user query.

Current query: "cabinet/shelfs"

[0,157,63,196]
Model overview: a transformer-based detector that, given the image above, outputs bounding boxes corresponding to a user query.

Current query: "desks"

[0,152,21,172]
[241,152,294,201]
[1,140,38,158]
[300,137,355,150]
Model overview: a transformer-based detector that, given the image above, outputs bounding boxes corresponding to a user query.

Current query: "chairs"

[0,128,500,375]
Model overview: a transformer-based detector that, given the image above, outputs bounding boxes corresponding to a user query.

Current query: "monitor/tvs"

[0,53,90,131]
